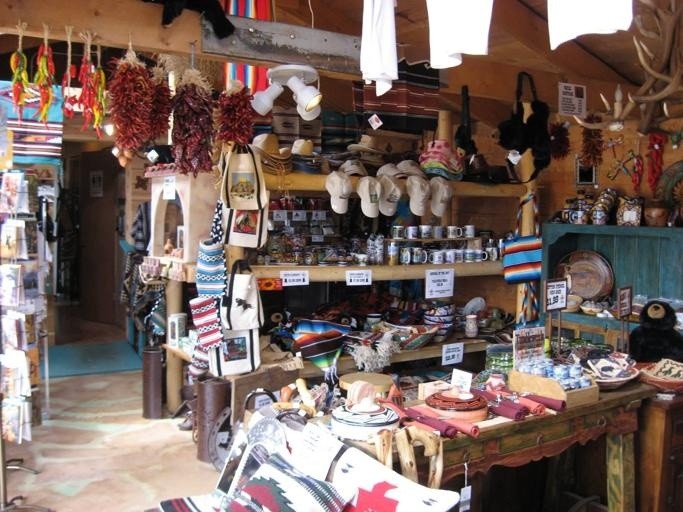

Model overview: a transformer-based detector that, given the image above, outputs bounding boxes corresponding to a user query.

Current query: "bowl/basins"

[462,296,488,318]
[423,301,457,344]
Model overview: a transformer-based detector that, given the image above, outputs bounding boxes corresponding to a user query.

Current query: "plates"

[579,300,604,316]
[560,293,583,313]
[596,360,642,390]
[553,248,615,300]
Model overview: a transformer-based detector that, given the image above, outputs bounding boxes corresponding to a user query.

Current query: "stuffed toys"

[628,300,683,362]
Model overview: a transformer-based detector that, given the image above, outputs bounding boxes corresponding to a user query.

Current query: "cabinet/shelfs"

[163,71,536,430]
[588,393,683,512]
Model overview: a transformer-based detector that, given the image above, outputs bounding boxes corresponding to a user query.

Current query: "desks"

[307,377,660,511]
[544,309,683,355]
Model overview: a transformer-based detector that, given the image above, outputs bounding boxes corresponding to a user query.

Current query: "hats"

[249,134,463,218]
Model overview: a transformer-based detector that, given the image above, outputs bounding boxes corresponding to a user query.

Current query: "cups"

[392,223,499,269]
[519,358,592,391]
[559,186,616,228]
[366,312,383,325]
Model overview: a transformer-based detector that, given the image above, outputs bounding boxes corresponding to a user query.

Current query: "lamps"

[251,63,325,122]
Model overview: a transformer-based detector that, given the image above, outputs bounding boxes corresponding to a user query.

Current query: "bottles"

[465,314,479,338]
[367,231,385,265]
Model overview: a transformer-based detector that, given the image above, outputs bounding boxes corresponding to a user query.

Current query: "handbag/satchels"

[188,145,270,378]
[455,70,551,184]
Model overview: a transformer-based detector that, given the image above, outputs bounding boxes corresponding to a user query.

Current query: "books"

[0,172,42,445]
